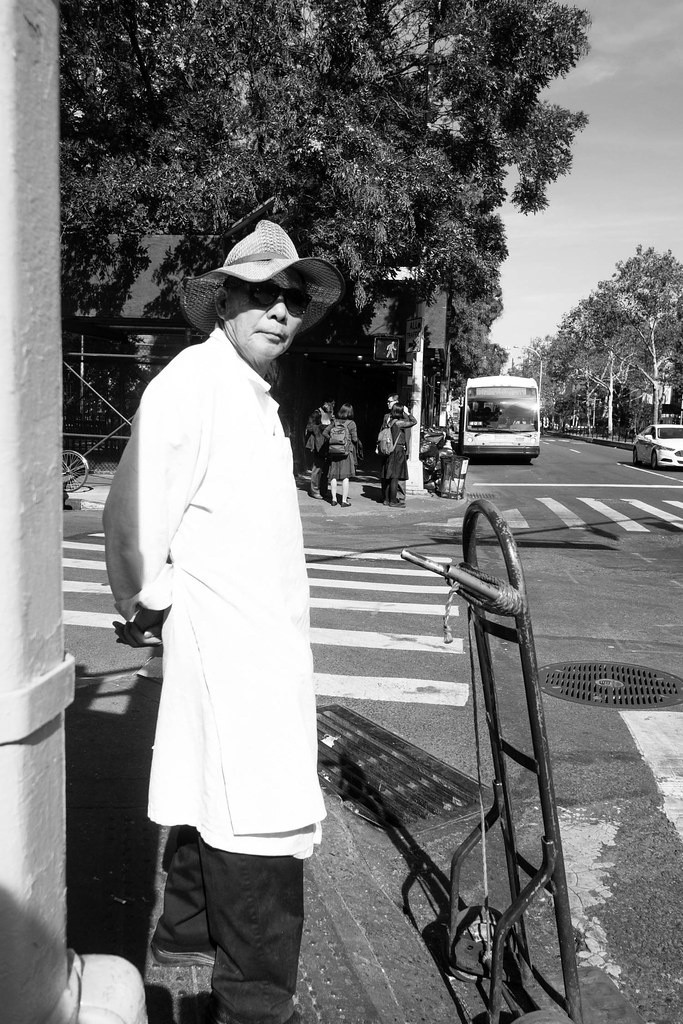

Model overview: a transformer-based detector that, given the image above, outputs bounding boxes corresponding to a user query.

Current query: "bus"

[459,376,540,462]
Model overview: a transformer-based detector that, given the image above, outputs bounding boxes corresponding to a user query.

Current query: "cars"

[633,424,683,470]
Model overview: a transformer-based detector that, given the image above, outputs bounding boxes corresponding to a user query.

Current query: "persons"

[322,403,358,507]
[100,220,346,1024]
[305,398,336,499]
[374,393,418,508]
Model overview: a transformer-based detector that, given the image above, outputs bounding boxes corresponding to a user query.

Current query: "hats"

[179,220,345,336]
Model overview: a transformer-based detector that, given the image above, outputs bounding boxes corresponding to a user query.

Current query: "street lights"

[514,345,542,403]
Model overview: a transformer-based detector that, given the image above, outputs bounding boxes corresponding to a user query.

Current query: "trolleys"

[402,498,584,1024]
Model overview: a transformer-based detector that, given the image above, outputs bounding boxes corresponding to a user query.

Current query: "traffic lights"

[373,337,399,363]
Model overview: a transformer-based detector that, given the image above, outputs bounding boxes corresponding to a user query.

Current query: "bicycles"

[63,450,89,492]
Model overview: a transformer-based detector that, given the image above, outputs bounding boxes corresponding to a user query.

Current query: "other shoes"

[341,502,351,507]
[151,941,217,967]
[331,501,337,506]
[383,498,401,507]
[207,1010,308,1024]
[320,491,332,497]
[308,490,323,499]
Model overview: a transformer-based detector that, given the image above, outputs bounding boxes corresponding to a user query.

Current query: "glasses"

[227,279,312,317]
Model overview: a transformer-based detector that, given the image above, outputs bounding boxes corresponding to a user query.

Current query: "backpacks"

[378,419,402,455]
[329,419,352,456]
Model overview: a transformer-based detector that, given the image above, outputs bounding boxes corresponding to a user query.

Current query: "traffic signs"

[406,317,422,352]
